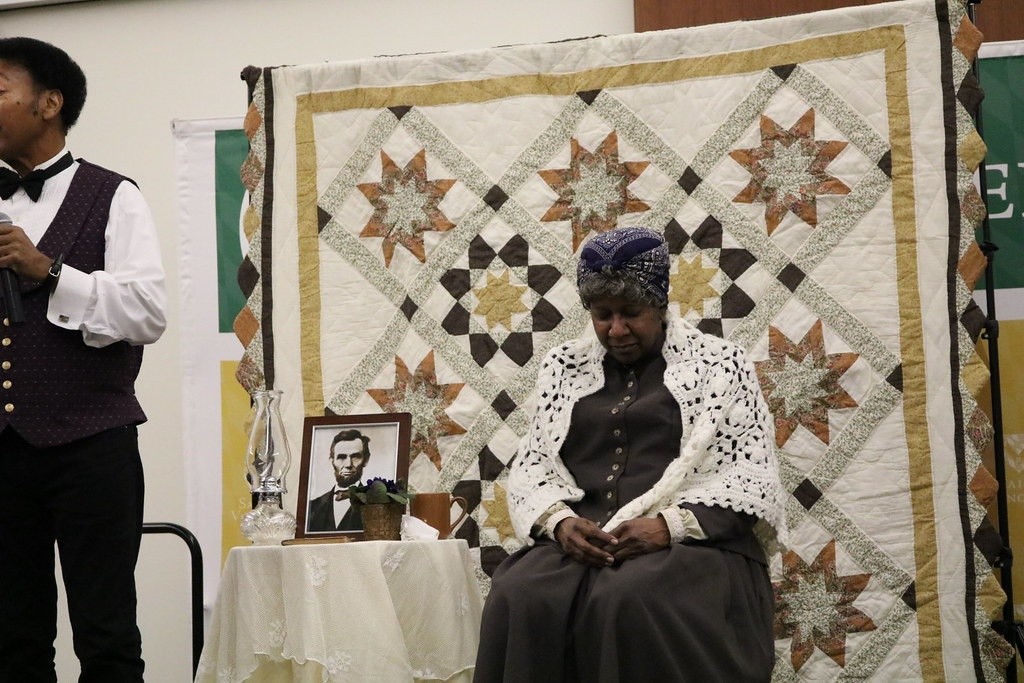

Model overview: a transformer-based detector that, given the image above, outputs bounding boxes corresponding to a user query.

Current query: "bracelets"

[35,251,65,290]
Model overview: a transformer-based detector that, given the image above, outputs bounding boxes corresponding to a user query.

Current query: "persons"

[306,428,370,531]
[471,225,786,683]
[1,36,166,683]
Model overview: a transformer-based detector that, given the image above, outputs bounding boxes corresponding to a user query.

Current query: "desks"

[195,537,487,683]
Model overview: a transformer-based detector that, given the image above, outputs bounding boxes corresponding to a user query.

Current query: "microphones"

[0,212,26,328]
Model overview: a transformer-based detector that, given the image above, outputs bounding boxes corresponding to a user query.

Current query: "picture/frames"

[293,410,412,540]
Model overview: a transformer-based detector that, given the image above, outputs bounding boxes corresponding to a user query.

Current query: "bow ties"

[0,148,74,202]
[333,489,354,502]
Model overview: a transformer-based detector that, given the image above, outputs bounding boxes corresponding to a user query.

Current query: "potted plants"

[345,480,418,539]
